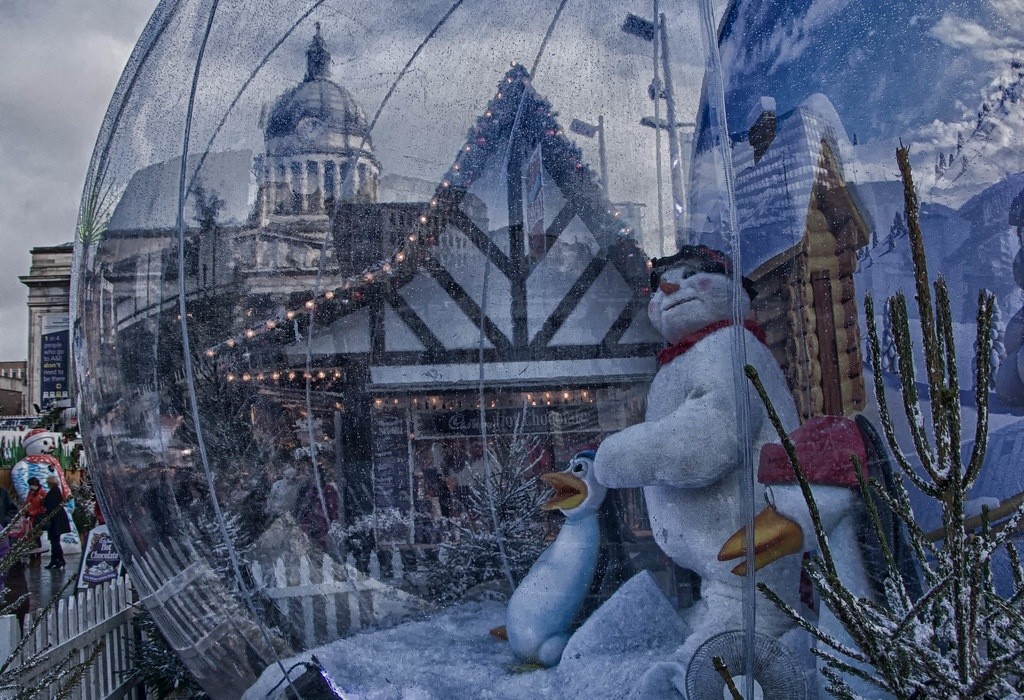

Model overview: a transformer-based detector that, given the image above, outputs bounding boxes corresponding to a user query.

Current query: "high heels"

[44,561,57,568]
[53,560,66,569]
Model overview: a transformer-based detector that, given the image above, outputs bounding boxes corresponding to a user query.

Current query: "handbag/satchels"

[32,512,53,531]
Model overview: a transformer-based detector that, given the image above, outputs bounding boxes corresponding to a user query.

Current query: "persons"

[81,416,366,572]
[0,474,108,643]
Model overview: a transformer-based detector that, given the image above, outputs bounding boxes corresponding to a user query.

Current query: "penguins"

[486,448,631,667]
[718,413,925,700]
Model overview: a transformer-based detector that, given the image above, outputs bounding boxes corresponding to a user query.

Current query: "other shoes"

[32,557,40,567]
[28,559,34,567]
